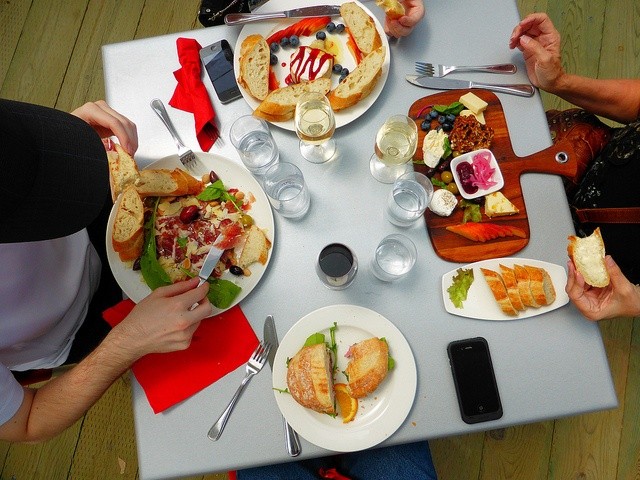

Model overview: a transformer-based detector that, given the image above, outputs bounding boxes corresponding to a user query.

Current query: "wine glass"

[292,91,337,165]
[314,242,359,292]
[368,113,419,185]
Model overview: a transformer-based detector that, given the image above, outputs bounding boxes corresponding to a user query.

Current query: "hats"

[1,99,108,245]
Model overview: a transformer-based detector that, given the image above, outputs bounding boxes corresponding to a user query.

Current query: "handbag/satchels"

[544,107,613,195]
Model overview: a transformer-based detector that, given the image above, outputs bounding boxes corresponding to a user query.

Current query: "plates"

[271,303,419,453]
[233,0,391,132]
[449,148,505,200]
[441,257,570,322]
[105,151,275,320]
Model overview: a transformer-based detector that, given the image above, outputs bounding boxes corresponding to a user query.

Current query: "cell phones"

[446,336,504,426]
[198,39,242,104]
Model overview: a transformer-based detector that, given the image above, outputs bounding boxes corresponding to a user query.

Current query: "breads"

[287,344,334,414]
[481,268,518,314]
[340,2,382,54]
[514,264,542,309]
[252,77,331,122]
[376,1,405,19]
[240,225,271,276]
[346,337,387,399]
[103,138,139,201]
[499,264,528,310]
[566,226,611,288]
[136,168,204,195]
[485,191,519,218]
[237,33,270,102]
[329,45,386,112]
[524,265,556,305]
[111,187,145,262]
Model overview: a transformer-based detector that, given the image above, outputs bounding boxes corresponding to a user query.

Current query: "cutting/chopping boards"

[407,89,578,264]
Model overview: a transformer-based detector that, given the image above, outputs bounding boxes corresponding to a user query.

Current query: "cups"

[382,171,434,228]
[369,233,419,283]
[228,114,280,176]
[262,161,311,221]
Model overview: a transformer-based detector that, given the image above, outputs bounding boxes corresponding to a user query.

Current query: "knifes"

[190,232,225,311]
[223,5,341,26]
[404,73,535,98]
[262,314,303,458]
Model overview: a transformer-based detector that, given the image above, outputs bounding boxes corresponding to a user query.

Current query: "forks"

[202,121,219,141]
[414,61,517,78]
[149,97,198,173]
[206,339,273,442]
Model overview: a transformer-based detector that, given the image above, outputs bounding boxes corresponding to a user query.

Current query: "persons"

[508,13,639,320]
[197,0,425,39]
[0,99,209,445]
